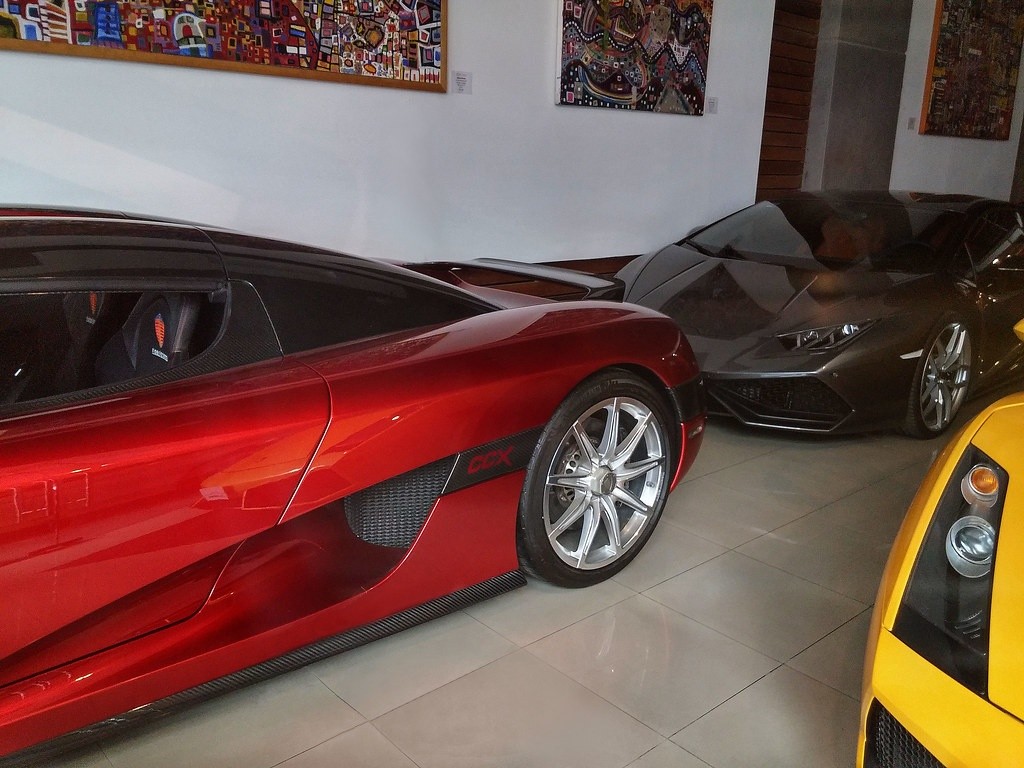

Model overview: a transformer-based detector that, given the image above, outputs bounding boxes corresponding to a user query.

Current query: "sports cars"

[0,202,708,768]
[614,192,1024,439]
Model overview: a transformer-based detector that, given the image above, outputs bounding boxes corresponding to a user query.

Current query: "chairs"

[813,207,884,261]
[93,290,201,386]
[2,290,116,407]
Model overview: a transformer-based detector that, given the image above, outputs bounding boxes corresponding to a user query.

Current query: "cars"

[857,317,1024,768]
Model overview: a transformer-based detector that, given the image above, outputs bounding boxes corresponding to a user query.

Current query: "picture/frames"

[0,0,448,94]
